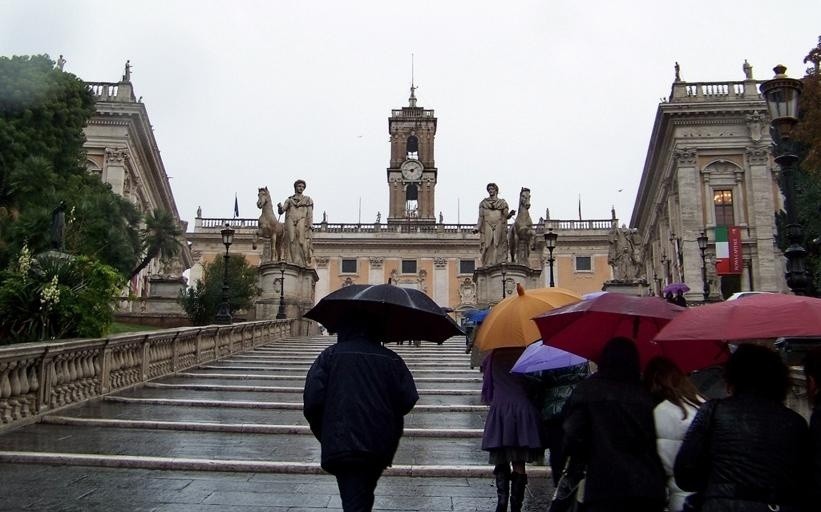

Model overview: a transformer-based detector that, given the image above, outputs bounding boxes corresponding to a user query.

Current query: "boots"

[510,471,528,512]
[493,465,511,511]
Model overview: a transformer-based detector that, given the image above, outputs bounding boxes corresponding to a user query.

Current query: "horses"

[253,186,285,262]
[508,187,536,264]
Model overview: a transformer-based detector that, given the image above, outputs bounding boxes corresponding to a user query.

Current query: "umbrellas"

[303,277,466,347]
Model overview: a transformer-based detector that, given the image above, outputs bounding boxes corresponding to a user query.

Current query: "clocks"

[401,159,423,180]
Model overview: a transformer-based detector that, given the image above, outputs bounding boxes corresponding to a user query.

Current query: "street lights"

[543,227,558,288]
[214,222,235,324]
[497,261,508,301]
[274,256,289,320]
[758,64,813,298]
[696,232,711,303]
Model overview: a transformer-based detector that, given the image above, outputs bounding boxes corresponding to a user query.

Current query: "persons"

[277,179,311,267]
[673,344,821,512]
[473,183,515,267]
[304,318,419,512]
[479,338,708,512]
[665,289,687,307]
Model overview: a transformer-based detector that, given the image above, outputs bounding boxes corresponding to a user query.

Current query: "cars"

[724,291,775,302]
